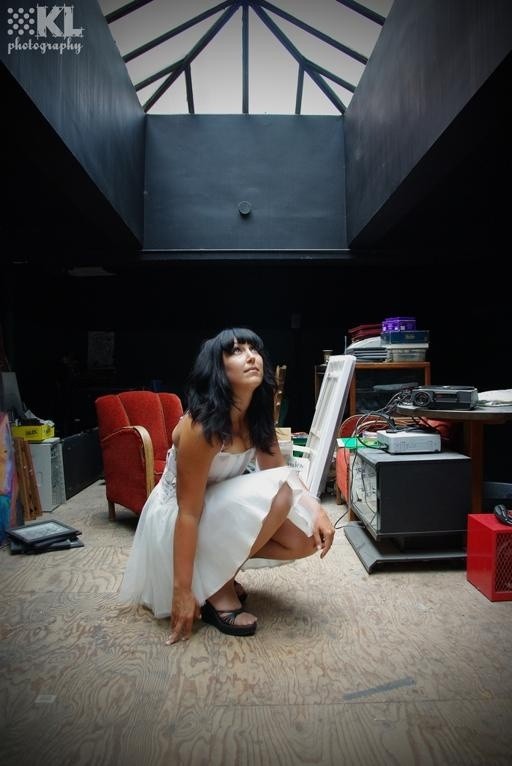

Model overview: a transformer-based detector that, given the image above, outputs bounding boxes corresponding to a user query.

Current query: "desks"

[396,402,512,514]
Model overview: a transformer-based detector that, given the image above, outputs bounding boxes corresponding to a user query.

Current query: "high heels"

[232,579,249,604]
[198,600,260,637]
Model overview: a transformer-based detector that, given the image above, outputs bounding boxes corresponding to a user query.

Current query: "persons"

[123,327,334,646]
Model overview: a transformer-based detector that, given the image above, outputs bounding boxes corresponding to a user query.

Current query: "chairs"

[335,414,455,522]
[95,390,184,521]
[273,365,287,426]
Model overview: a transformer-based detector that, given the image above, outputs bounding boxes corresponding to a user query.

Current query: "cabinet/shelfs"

[314,361,431,418]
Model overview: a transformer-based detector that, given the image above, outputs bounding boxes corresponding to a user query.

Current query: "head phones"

[494,505,512,526]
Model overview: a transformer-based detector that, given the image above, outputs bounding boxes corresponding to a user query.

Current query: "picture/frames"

[5,519,84,555]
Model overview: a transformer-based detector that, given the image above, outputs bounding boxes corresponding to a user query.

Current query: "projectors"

[411,386,478,410]
[377,429,442,454]
